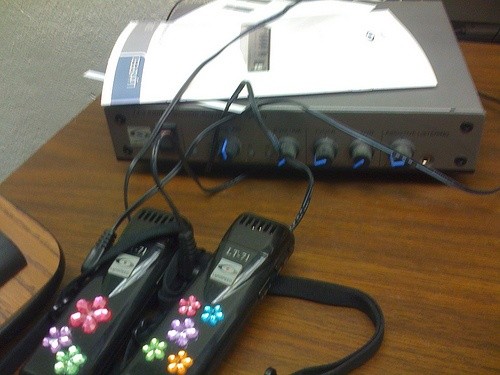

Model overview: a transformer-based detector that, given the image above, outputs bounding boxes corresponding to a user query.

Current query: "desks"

[0,29,500,375]
